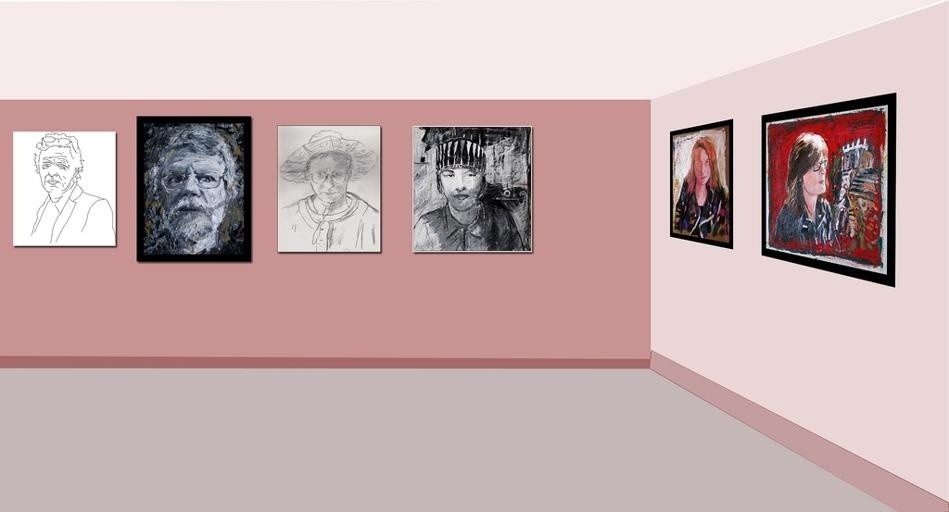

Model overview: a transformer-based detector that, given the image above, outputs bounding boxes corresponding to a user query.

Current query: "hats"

[279,130,377,183]
[419,127,517,179]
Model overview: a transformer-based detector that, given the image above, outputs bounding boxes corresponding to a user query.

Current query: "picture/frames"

[134,113,254,265]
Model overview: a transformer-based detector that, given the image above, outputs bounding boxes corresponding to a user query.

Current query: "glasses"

[813,159,828,171]
[161,171,223,190]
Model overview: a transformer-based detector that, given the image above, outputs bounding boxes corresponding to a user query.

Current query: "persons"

[20,131,115,247]
[667,135,732,241]
[771,131,839,248]
[414,161,529,251]
[280,147,379,250]
[141,125,246,255]
